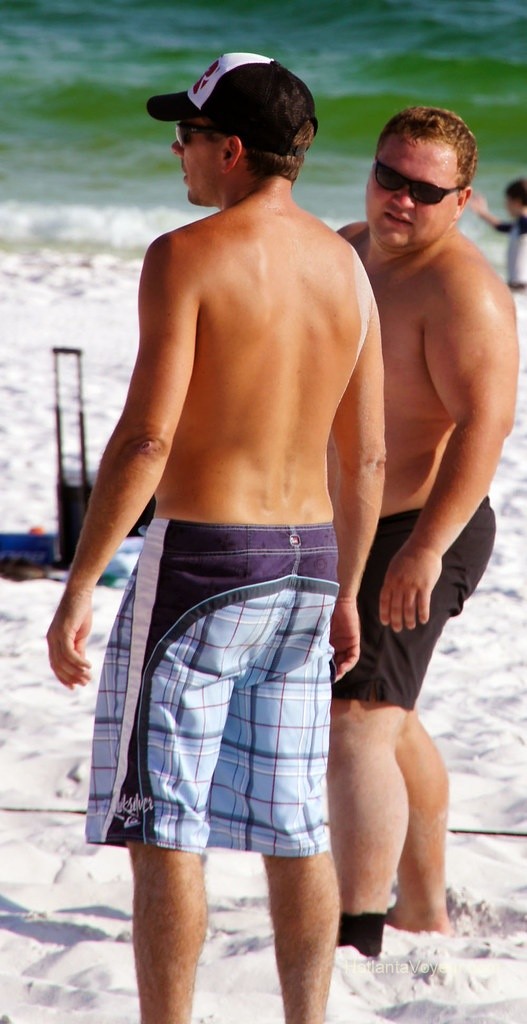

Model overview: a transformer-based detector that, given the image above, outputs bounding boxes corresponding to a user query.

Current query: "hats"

[147,54,317,156]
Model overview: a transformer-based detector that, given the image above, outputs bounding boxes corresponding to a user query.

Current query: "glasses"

[371,157,462,205]
[172,122,217,146]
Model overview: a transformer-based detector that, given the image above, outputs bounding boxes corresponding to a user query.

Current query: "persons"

[463,174,527,321]
[38,48,393,1024]
[295,97,522,963]
